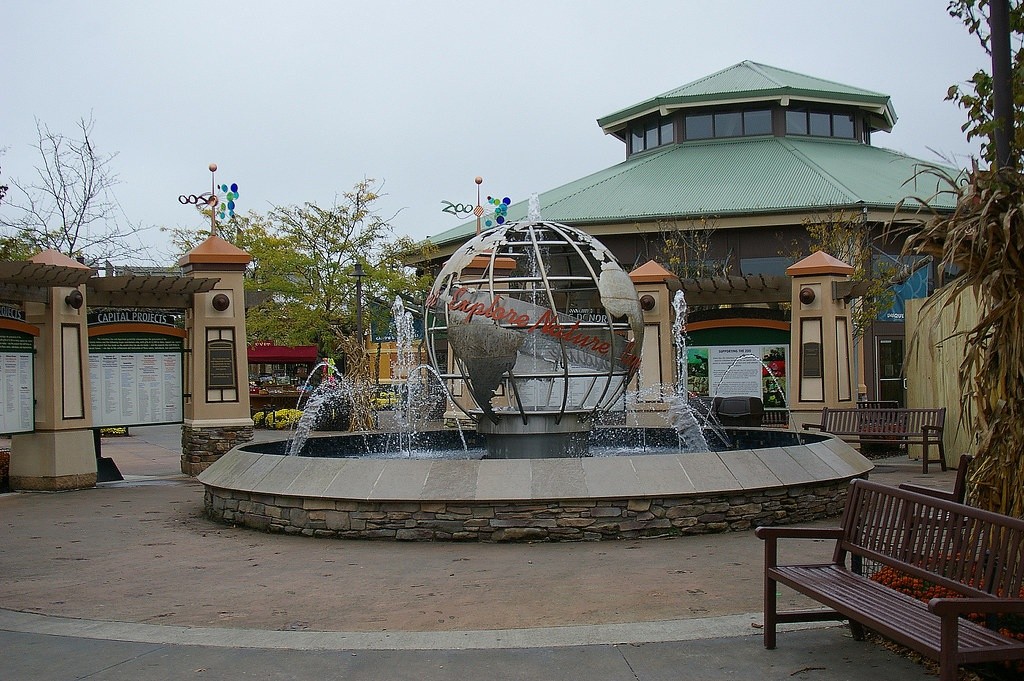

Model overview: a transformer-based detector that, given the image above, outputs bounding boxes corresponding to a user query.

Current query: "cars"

[258,372,274,385]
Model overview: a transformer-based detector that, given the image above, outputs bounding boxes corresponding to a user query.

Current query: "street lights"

[348,264,369,361]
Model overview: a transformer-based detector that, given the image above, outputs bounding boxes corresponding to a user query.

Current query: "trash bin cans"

[717,395,766,426]
[688,395,724,424]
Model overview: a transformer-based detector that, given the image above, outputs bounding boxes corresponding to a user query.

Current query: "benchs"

[755,478,1024,681]
[899,454,976,557]
[802,406,947,474]
